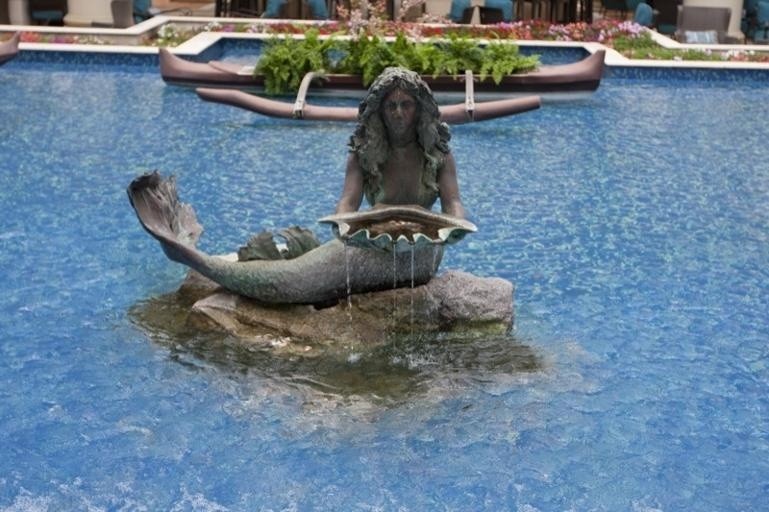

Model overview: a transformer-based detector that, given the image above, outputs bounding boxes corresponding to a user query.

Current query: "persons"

[332,64,470,242]
